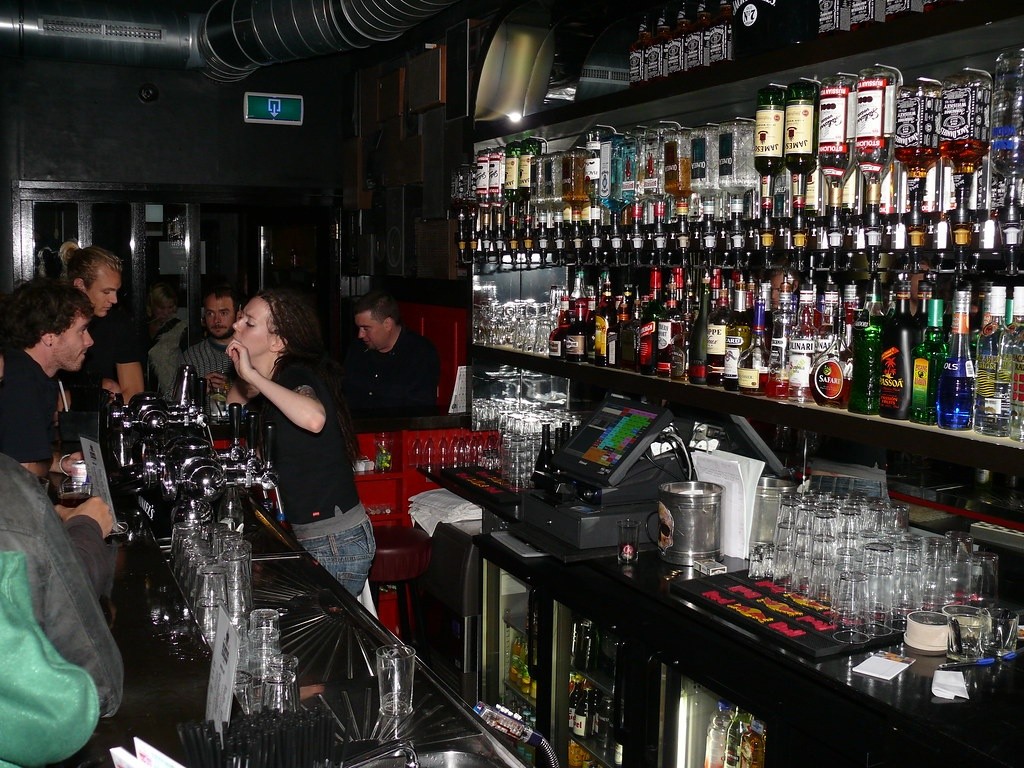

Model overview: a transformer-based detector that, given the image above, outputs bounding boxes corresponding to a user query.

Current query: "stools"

[365,526,432,679]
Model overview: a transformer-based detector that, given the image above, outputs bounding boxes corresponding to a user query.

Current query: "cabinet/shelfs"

[471,1,1024,472]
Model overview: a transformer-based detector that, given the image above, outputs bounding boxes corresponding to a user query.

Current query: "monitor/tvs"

[550,395,675,486]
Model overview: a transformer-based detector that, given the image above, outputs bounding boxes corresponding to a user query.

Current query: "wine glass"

[411,430,496,467]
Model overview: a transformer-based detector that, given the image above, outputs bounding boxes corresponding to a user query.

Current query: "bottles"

[209,387,227,415]
[375,433,392,471]
[536,424,552,472]
[509,616,626,768]
[197,376,208,412]
[772,46,1024,268]
[549,269,836,394]
[554,427,564,453]
[703,700,764,768]
[843,274,1024,441]
[561,422,571,445]
[754,86,786,215]
[218,488,244,539]
[629,0,732,86]
[810,305,853,408]
[448,127,760,268]
[572,425,581,438]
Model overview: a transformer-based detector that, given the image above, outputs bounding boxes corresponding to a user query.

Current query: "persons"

[0,451,121,768]
[0,240,446,603]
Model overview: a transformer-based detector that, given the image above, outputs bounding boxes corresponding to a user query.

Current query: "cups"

[213,369,231,392]
[473,395,581,430]
[474,292,548,355]
[945,614,986,662]
[170,523,301,714]
[171,364,197,407]
[58,483,92,508]
[376,644,415,717]
[499,430,542,489]
[979,608,1017,655]
[750,492,998,644]
[617,520,640,563]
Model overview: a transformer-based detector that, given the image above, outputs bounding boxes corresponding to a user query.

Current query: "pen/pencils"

[1002,646,1024,659]
[938,657,994,668]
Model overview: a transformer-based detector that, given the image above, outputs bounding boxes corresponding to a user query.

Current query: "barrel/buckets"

[658,482,725,565]
